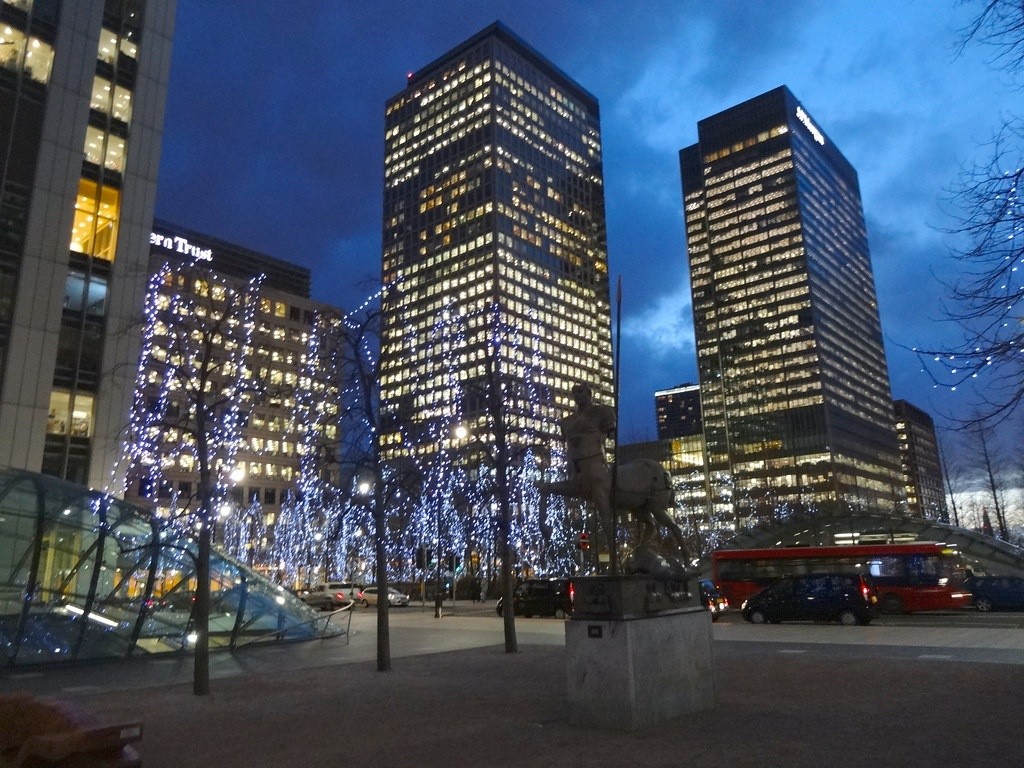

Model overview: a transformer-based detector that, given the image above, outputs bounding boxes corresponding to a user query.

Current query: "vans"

[739,570,882,627]
[495,577,575,619]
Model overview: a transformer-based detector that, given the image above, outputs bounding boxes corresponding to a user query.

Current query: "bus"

[707,543,974,616]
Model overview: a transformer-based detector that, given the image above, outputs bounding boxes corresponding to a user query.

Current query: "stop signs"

[578,533,591,549]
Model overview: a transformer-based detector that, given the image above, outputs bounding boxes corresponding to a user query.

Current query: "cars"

[129,583,285,617]
[360,588,410,608]
[300,588,338,610]
[699,579,730,622]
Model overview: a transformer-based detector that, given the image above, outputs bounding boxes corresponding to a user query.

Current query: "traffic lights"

[415,547,424,570]
[449,555,454,573]
[454,555,462,569]
[425,548,434,569]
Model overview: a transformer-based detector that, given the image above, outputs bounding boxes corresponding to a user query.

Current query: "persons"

[549,383,620,574]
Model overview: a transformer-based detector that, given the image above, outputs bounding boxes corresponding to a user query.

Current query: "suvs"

[963,576,1024,612]
[317,583,362,602]
